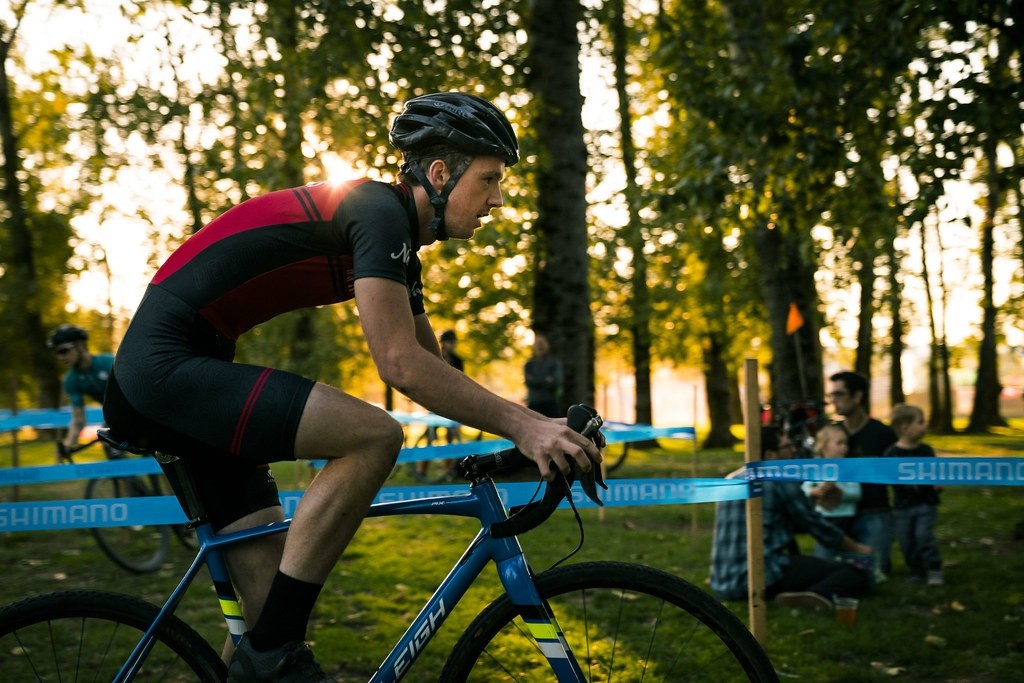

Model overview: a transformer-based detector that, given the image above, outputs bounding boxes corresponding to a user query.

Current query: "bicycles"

[55,439,203,574]
[1,403,781,683]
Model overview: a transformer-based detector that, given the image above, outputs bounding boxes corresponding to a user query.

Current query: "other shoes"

[775,591,832,616]
[927,569,944,585]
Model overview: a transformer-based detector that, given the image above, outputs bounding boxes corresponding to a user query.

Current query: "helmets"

[45,326,89,349]
[439,330,456,342]
[388,91,519,167]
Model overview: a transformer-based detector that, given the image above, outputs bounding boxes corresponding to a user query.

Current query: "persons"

[706,425,879,618]
[797,426,861,565]
[426,329,464,443]
[106,92,603,683]
[879,404,945,586]
[813,370,899,587]
[48,326,152,498]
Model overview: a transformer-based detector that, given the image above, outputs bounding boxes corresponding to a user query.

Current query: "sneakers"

[227,633,331,683]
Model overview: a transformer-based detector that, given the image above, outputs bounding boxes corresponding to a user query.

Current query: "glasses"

[776,441,795,450]
[56,345,76,356]
[831,390,851,397]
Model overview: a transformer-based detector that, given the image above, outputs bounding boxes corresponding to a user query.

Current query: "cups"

[833,598,860,626]
